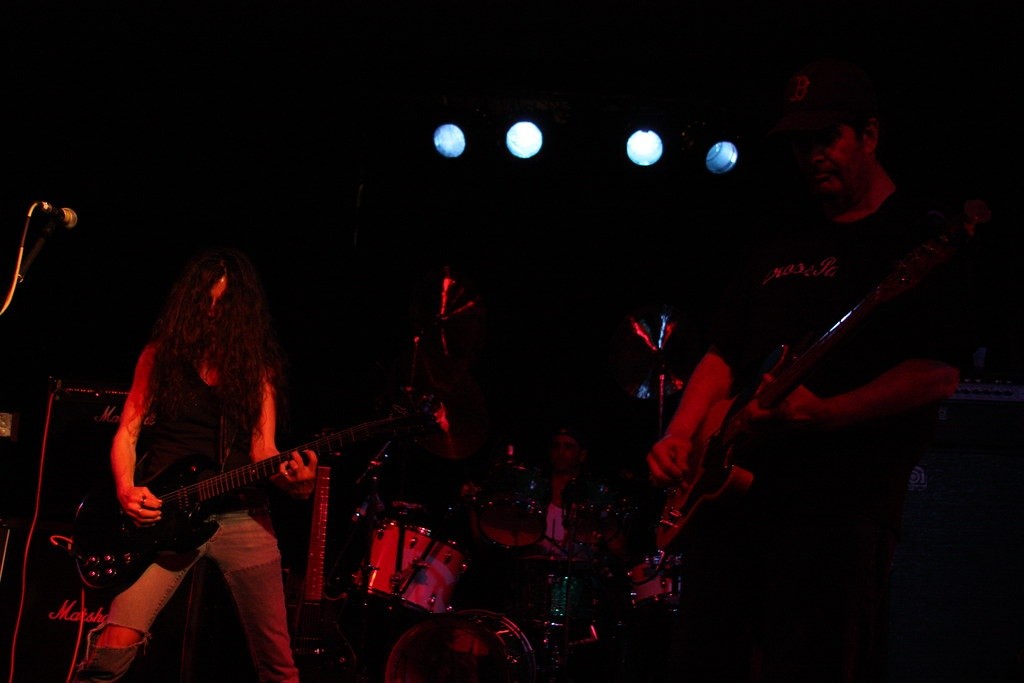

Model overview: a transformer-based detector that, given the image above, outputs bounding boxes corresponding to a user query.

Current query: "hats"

[774,59,878,141]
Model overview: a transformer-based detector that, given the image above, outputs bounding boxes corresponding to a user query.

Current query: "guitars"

[65,401,447,596]
[649,192,999,562]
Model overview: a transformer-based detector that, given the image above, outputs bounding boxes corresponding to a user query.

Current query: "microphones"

[35,201,77,228]
[47,376,71,400]
[356,439,392,486]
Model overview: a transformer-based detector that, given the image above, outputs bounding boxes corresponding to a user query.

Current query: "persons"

[76,251,321,683]
[644,60,968,683]
[461,423,625,551]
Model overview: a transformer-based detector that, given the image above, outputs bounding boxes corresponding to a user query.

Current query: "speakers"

[0,388,289,683]
[880,446,1024,683]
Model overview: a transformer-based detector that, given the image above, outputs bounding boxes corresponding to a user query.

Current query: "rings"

[282,471,288,478]
[140,497,147,508]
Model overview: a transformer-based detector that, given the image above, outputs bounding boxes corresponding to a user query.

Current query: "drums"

[380,597,566,683]
[343,508,480,627]
